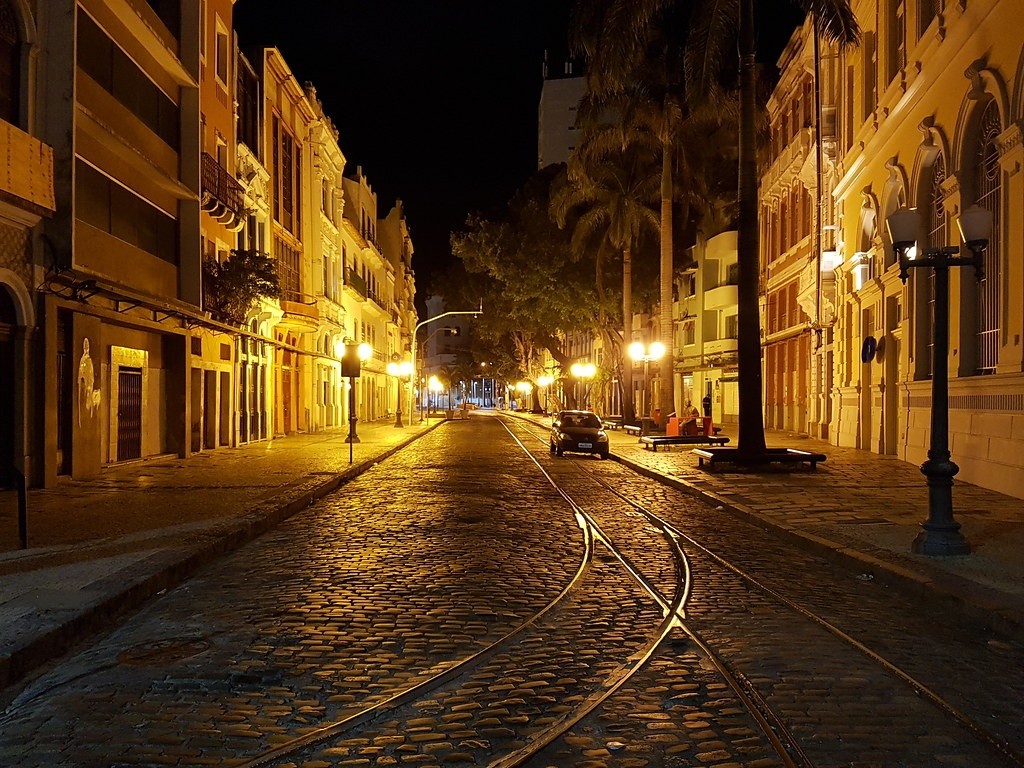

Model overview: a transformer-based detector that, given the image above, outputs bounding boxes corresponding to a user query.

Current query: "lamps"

[803,327,823,355]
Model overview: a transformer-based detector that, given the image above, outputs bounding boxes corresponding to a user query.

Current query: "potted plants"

[438,365,461,419]
[459,363,478,419]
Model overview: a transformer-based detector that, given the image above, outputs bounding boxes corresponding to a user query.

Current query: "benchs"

[512,408,826,473]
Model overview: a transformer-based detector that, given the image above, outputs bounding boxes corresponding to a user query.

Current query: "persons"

[702,394,711,416]
[679,398,700,436]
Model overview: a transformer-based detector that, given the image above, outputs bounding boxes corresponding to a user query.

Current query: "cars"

[549,410,610,461]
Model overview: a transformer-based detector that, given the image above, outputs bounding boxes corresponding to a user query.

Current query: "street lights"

[336,338,372,444]
[628,341,666,443]
[517,382,530,413]
[570,363,597,411]
[538,376,555,417]
[884,201,994,558]
[387,361,411,428]
